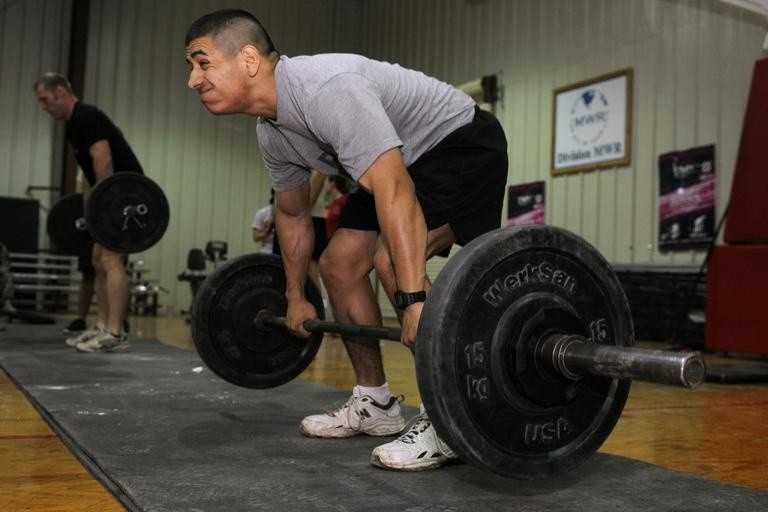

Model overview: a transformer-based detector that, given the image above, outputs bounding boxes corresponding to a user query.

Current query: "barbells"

[182,223,704,485]
[44,170,171,255]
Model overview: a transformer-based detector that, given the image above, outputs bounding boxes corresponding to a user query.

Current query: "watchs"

[393,289,428,311]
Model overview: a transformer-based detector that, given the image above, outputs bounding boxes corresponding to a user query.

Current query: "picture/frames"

[549,66,634,177]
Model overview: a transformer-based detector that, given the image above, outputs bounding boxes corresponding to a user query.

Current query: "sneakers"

[67,323,104,348]
[76,329,130,354]
[297,387,406,438]
[370,412,459,471]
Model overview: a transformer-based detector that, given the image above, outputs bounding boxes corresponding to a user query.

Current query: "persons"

[32,71,146,353]
[184,8,508,471]
[251,171,353,339]
[62,250,130,339]
